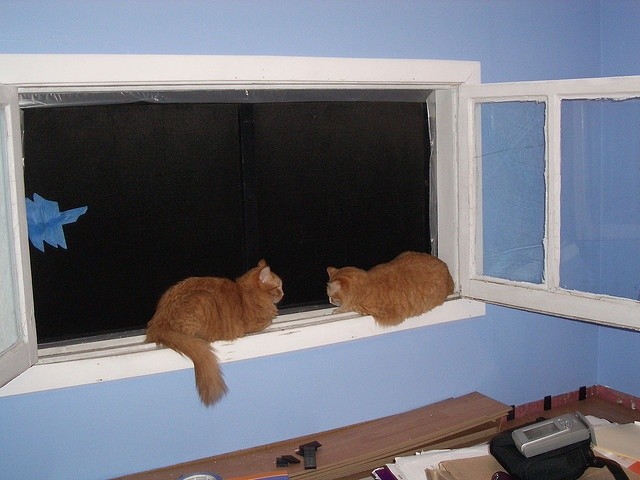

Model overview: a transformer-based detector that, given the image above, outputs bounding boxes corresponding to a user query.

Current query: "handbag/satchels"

[489,417,631,479]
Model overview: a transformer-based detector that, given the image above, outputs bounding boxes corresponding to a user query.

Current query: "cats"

[142,258,285,410]
[326,250,457,330]
